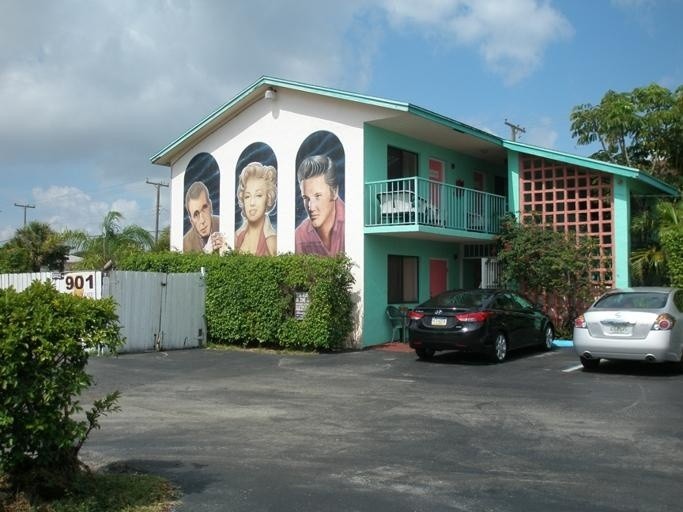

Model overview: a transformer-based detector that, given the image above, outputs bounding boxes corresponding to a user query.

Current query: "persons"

[234,162,278,257]
[293,153,345,259]
[182,180,227,256]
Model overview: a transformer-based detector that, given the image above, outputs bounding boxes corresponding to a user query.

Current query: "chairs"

[386,304,410,344]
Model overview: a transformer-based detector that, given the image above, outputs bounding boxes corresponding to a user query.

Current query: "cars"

[573,286,683,369]
[408,289,554,363]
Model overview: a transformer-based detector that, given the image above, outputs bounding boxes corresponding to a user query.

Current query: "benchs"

[377,190,494,233]
